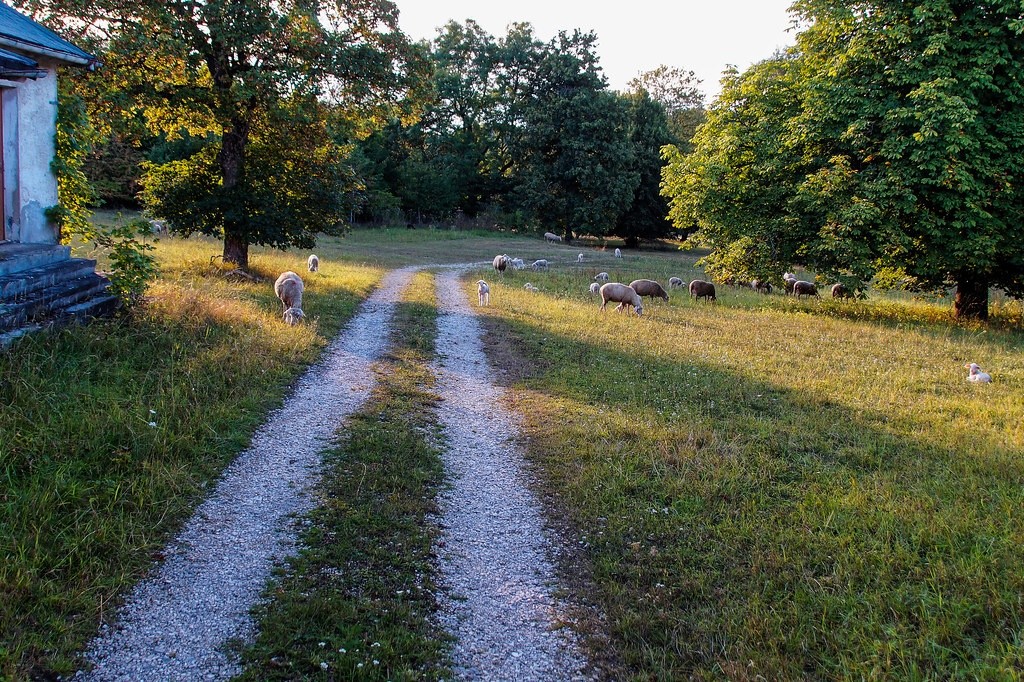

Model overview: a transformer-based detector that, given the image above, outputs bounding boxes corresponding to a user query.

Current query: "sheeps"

[615,248,621,257]
[476,280,490,306]
[493,254,507,274]
[595,272,608,283]
[544,232,562,244]
[785,278,798,296]
[964,363,993,384]
[589,282,600,294]
[688,279,717,305]
[599,282,643,317]
[503,253,525,270]
[577,254,583,262]
[831,283,856,304]
[308,254,319,272]
[274,271,307,327]
[524,283,539,292]
[783,270,797,280]
[724,276,772,294]
[532,259,548,271]
[629,279,670,303]
[669,277,687,290]
[793,280,821,302]
[149,220,168,240]
